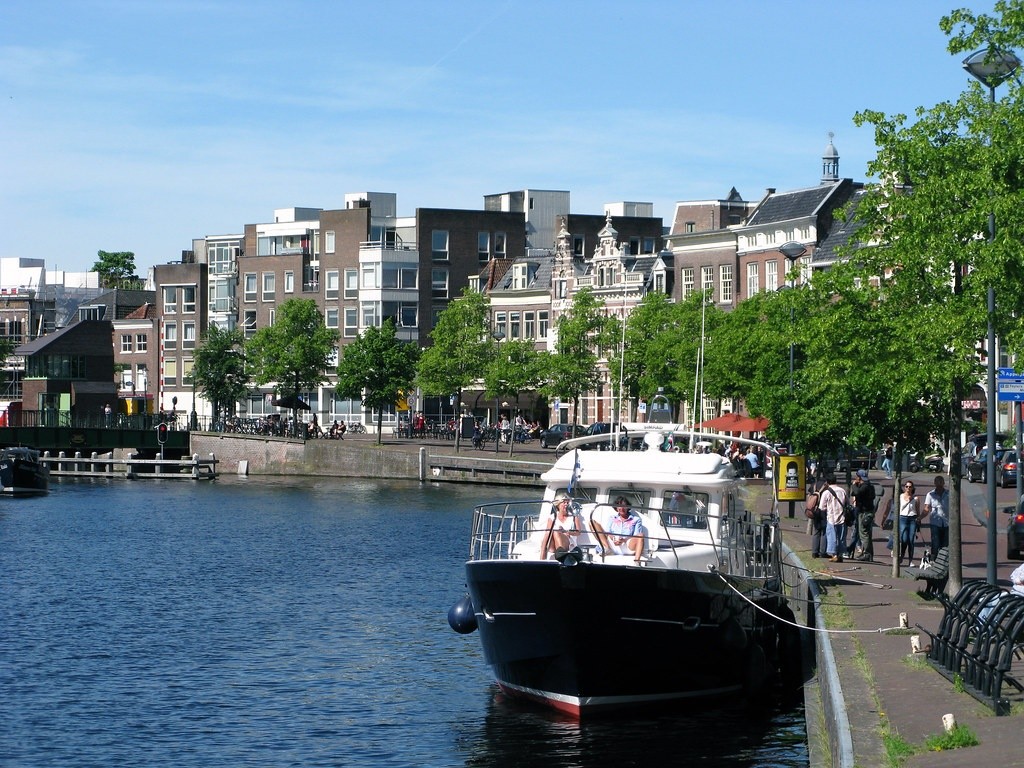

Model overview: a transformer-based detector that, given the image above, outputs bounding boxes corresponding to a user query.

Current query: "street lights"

[780,243,806,516]
[493,332,506,453]
[189,366,199,430]
[142,366,150,416]
[964,57,1018,592]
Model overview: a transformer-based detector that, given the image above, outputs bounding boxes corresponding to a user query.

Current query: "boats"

[452,283,811,718]
[0,449,51,496]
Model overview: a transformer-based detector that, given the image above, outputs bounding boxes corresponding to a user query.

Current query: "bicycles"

[473,423,530,450]
[348,423,366,434]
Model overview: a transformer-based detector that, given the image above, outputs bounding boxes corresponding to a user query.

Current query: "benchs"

[326,428,346,440]
[904,546,949,601]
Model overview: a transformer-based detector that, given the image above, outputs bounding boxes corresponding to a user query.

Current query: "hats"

[856,469,870,482]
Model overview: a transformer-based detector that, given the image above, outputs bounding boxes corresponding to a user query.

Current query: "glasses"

[906,486,912,488]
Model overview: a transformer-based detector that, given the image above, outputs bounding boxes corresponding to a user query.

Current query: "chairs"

[392,427,461,441]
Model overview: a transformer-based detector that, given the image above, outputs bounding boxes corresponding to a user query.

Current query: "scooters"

[909,451,943,473]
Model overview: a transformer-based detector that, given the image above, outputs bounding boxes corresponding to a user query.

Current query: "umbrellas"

[272,395,311,410]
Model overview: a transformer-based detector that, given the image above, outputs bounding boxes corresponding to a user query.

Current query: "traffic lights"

[158,423,169,445]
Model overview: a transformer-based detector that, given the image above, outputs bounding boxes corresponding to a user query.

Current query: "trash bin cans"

[302,423,308,439]
[462,416,483,438]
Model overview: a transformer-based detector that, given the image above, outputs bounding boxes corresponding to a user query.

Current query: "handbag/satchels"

[883,520,894,530]
[846,507,855,527]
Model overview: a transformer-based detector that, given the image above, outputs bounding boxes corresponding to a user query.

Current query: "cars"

[1002,497,1023,560]
[836,446,879,472]
[997,452,1024,489]
[540,424,584,448]
[587,423,617,450]
[968,447,1002,482]
[959,441,981,478]
[216,415,294,433]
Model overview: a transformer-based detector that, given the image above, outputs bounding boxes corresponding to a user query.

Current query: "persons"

[916,476,949,562]
[1010,563,1024,597]
[807,467,834,559]
[540,492,581,563]
[44,402,50,426]
[492,413,545,444]
[160,406,180,429]
[257,412,286,438]
[882,440,894,479]
[882,486,904,557]
[589,495,644,566]
[312,412,319,439]
[899,481,920,567]
[216,407,242,434]
[308,421,314,434]
[447,417,463,439]
[851,469,876,562]
[819,473,850,562]
[329,420,339,439]
[719,442,761,478]
[413,411,431,438]
[105,404,111,428]
[472,420,484,449]
[332,420,347,439]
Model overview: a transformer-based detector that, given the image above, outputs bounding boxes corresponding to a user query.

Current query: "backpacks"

[805,481,827,519]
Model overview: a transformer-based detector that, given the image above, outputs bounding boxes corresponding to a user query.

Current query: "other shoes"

[599,548,610,557]
[857,554,873,561]
[829,555,843,562]
[886,476,892,480]
[812,552,819,558]
[843,552,853,559]
[820,553,832,558]
[908,561,916,567]
[899,553,905,563]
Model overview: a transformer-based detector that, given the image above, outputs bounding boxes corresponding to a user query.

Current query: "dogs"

[918,550,936,571]
